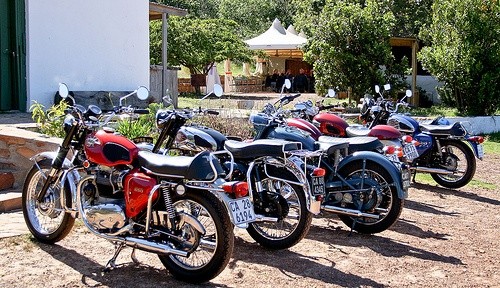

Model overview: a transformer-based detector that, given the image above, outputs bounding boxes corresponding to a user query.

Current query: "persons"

[264,68,316,93]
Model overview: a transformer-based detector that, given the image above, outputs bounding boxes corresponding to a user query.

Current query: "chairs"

[269,75,316,93]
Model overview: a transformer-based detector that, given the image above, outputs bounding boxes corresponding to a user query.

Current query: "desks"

[235,78,265,92]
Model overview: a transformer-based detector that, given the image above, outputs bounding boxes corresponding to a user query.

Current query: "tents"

[238,18,312,52]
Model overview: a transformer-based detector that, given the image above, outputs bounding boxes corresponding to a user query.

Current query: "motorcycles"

[123,84,330,250]
[21,83,251,285]
[242,79,411,233]
[288,82,488,189]
